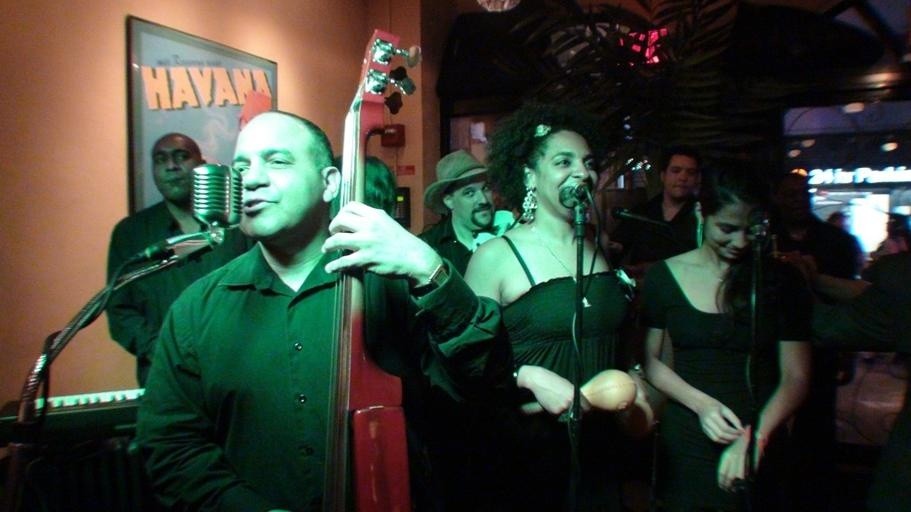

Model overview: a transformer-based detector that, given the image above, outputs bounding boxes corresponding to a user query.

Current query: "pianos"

[2,388,143,436]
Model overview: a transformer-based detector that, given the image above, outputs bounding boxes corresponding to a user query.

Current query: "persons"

[417,100,911,511]
[107,97,511,510]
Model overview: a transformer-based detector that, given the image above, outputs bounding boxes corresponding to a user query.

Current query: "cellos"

[322,26,423,512]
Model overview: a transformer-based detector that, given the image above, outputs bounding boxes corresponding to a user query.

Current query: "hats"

[422,149,490,215]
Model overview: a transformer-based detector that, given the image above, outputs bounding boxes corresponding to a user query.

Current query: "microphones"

[558,182,594,210]
[608,204,668,230]
[745,204,767,266]
[142,159,245,261]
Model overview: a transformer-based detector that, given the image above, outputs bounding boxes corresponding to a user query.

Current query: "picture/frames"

[125,15,277,216]
